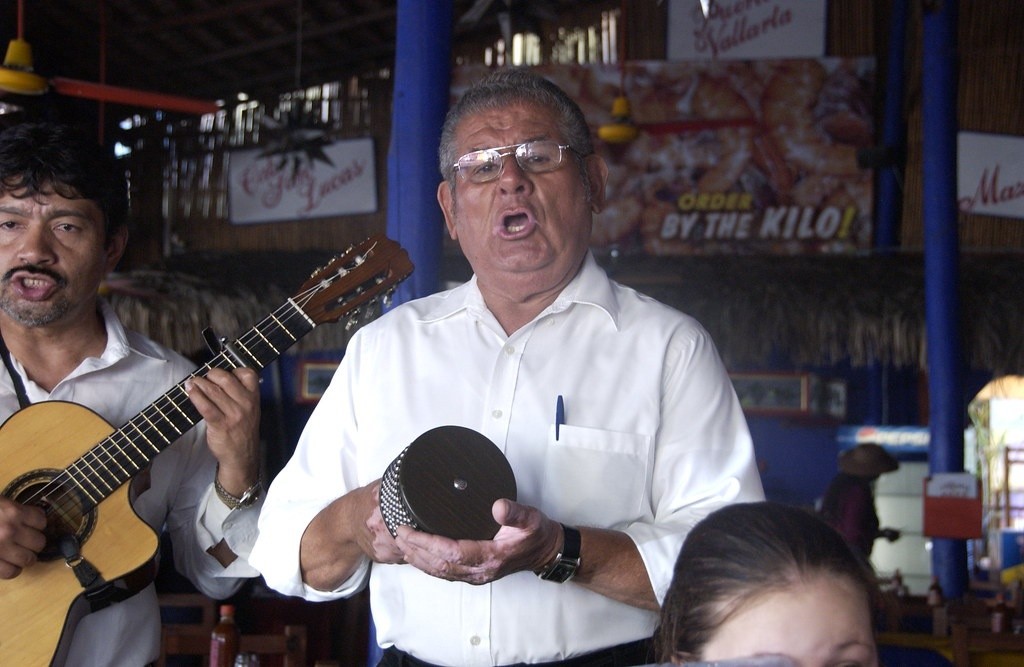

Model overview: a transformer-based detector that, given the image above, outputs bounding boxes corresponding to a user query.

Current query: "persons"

[823,442,903,564]
[0,107,269,667]
[653,499,882,667]
[249,67,770,667]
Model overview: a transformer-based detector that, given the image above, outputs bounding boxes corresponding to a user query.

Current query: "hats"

[838,443,900,473]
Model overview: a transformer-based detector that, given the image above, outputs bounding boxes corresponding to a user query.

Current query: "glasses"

[452,139,579,184]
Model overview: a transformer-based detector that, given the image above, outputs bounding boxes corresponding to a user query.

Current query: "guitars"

[0,231,417,667]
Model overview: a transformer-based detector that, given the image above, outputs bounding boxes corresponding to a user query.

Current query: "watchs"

[214,460,264,511]
[532,522,583,585]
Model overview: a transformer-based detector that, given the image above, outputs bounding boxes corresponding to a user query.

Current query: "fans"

[0,1,222,116]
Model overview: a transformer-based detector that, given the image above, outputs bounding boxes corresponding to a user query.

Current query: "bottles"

[209,605,238,667]
